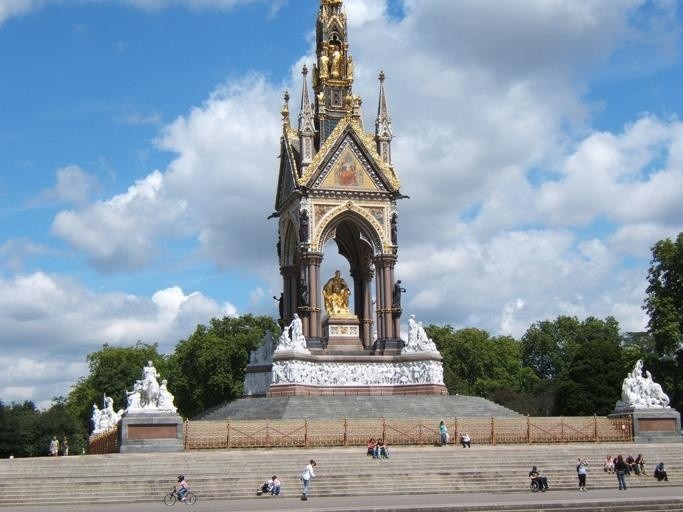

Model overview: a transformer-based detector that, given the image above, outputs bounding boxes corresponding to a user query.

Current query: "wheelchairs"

[527,475,547,492]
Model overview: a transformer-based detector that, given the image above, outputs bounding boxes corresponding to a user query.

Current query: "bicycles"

[163,487,195,507]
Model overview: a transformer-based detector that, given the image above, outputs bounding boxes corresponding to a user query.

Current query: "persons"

[269,474,281,496]
[367,436,378,458]
[176,475,188,502]
[89,360,178,433]
[393,279,405,306]
[576,457,590,492]
[49,436,59,456]
[243,312,445,396]
[459,432,471,448]
[528,465,550,489]
[322,270,351,318]
[603,453,670,491]
[298,459,319,502]
[273,292,283,319]
[439,420,448,447]
[374,438,391,459]
[621,359,670,408]
[60,436,69,455]
